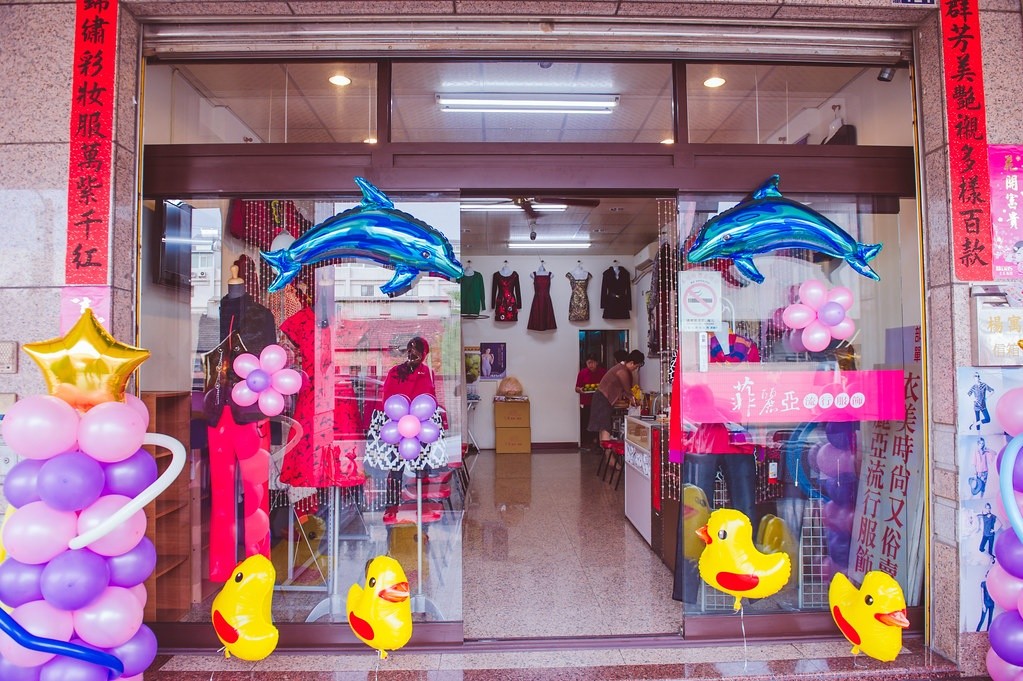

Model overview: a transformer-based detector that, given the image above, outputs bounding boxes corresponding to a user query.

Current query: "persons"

[460,262,631,330]
[670,320,760,603]
[575,350,645,472]
[192,264,282,583]
[382,337,453,535]
[647,235,670,359]
[482,346,494,377]
[967,372,1002,631]
[279,267,371,488]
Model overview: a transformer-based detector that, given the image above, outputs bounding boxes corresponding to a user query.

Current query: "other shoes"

[416,507,440,521]
[381,506,397,522]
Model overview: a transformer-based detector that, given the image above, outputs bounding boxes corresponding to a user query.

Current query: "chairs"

[596,439,623,477]
[347,429,471,623]
[609,446,625,491]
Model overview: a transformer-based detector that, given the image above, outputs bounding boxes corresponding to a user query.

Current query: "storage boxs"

[494,401,531,428]
[496,428,532,453]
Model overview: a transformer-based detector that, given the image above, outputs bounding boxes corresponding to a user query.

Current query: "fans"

[464,196,601,218]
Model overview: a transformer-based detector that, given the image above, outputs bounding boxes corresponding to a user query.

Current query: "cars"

[334,374,450,440]
[682,405,753,445]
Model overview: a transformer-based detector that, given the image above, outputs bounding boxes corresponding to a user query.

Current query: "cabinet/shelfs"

[623,415,679,571]
[138,389,225,623]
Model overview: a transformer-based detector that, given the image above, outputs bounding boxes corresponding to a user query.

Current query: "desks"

[466,400,480,454]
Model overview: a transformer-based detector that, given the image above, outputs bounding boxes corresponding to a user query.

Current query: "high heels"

[605,459,622,471]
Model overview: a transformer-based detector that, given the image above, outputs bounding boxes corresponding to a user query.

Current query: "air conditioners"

[633,241,659,271]
[198,271,206,278]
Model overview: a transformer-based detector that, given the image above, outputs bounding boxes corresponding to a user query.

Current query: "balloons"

[985,386,1023,681]
[347,555,412,660]
[829,570,909,661]
[0,309,186,681]
[694,507,791,613]
[781,280,856,351]
[258,175,464,294]
[382,394,440,460]
[211,554,279,661]
[686,173,883,285]
[809,383,861,579]
[684,483,804,589]
[231,345,303,416]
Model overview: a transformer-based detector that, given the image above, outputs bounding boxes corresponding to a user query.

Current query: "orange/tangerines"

[582,383,600,391]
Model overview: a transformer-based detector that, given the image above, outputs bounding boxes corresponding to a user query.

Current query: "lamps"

[441,105,614,115]
[435,93,620,108]
